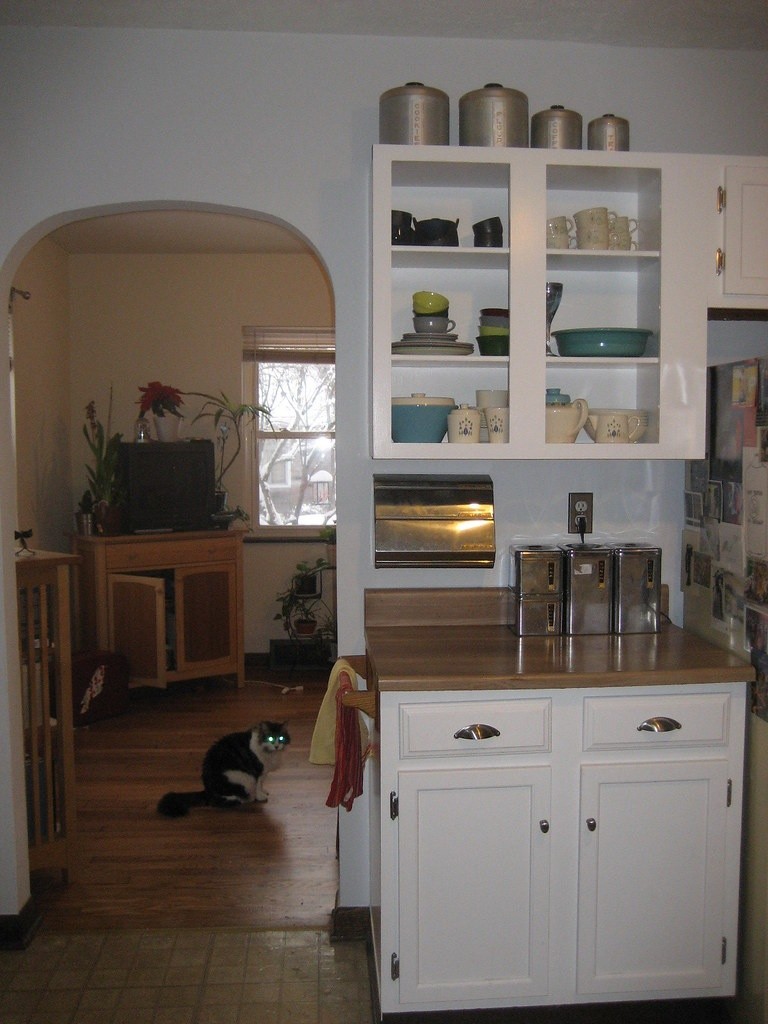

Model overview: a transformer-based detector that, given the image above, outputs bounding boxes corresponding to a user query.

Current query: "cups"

[476,389,509,428]
[484,407,508,442]
[472,217,504,248]
[547,207,639,252]
[545,282,563,357]
[391,209,412,248]
[412,291,456,333]
[447,404,480,443]
[546,400,588,443]
[588,413,640,443]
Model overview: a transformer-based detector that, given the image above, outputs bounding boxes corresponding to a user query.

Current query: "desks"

[15,546,84,889]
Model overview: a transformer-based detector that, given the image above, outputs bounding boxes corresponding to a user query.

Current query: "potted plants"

[185,388,279,534]
[82,420,129,535]
[273,522,337,661]
[75,491,99,536]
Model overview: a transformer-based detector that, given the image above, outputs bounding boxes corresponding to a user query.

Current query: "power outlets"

[568,490,592,534]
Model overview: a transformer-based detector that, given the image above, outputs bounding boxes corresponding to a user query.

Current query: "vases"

[151,415,183,443]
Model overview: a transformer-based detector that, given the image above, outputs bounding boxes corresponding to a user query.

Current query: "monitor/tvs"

[118,441,216,534]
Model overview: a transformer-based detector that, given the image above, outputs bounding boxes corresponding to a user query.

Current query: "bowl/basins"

[583,408,647,443]
[412,216,459,247]
[551,327,654,357]
[476,309,509,356]
[391,394,458,442]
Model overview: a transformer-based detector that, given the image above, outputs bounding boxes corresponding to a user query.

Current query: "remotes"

[134,528,172,534]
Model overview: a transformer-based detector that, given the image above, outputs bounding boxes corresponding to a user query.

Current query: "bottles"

[546,389,570,406]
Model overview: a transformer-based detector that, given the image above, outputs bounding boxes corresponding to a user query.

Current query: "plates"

[391,333,474,356]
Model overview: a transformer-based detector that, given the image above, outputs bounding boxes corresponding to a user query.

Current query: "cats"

[157,719,290,817]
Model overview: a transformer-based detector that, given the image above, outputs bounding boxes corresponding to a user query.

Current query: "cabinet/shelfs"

[361,623,758,1021]
[715,155,768,312]
[371,143,715,460]
[68,529,249,690]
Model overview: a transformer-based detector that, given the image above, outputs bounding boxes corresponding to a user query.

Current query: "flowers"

[139,380,185,421]
